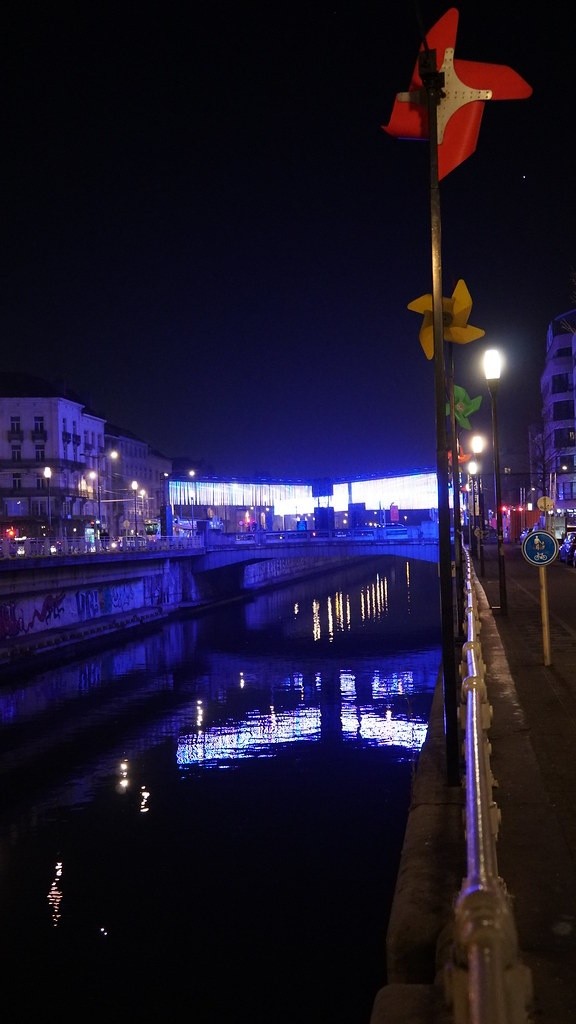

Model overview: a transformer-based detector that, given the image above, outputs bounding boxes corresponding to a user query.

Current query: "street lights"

[479,347,509,617]
[467,433,488,576]
[130,481,139,536]
[79,449,118,539]
[43,466,53,537]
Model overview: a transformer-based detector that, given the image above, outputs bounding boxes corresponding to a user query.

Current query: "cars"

[558,532,576,565]
